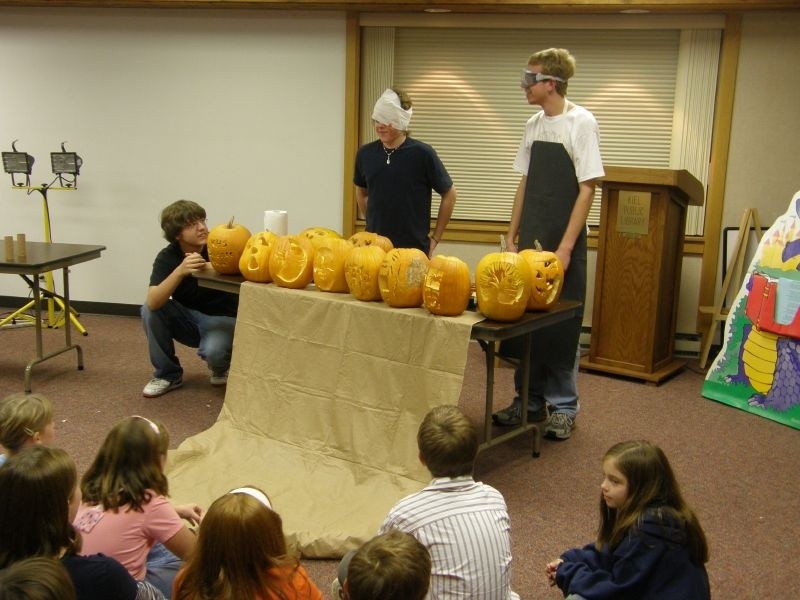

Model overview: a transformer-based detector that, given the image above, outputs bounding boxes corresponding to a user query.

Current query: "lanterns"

[206,216,565,319]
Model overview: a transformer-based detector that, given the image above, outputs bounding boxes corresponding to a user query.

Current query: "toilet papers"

[263,209,289,236]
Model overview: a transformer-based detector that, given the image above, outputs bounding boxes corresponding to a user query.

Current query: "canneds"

[4,236,14,259]
[16,234,26,256]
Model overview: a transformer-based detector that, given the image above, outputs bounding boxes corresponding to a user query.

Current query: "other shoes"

[331,578,346,600]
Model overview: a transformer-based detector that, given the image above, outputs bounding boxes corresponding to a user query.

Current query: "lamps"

[0,138,88,336]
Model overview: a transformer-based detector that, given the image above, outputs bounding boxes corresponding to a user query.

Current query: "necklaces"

[380,136,406,164]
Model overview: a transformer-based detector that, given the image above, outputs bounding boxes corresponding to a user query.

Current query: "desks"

[0,240,105,394]
[198,272,584,482]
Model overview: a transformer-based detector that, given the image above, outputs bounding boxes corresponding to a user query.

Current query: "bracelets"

[432,236,438,244]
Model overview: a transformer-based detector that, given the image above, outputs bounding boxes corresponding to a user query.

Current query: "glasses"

[522,69,564,87]
[183,218,208,229]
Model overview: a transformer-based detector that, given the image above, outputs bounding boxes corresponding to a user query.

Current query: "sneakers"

[210,367,229,384]
[143,374,183,397]
[492,399,546,425]
[544,412,575,440]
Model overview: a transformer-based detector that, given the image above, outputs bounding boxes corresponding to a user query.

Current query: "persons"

[491,47,605,441]
[544,440,712,600]
[140,200,235,397]
[71,414,206,598]
[349,87,456,261]
[170,485,326,600]
[342,530,432,600]
[0,393,138,600]
[336,405,521,600]
